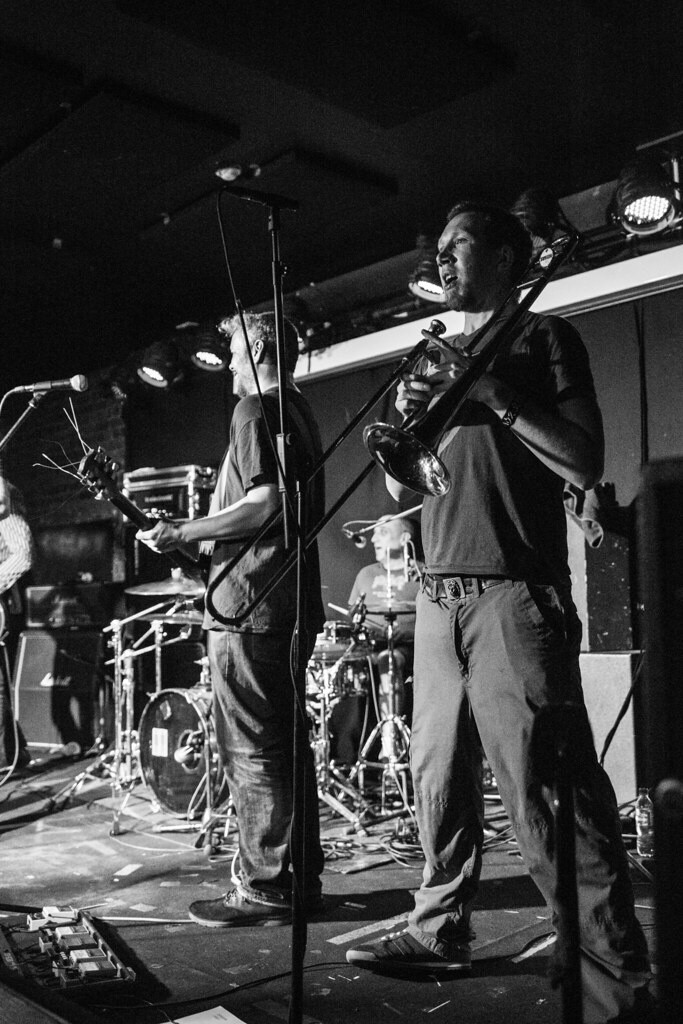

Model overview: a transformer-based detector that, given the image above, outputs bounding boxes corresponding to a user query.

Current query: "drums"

[137,686,231,819]
[306,632,372,698]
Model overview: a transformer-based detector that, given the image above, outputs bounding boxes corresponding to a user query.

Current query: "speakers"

[12,629,132,752]
[579,650,647,818]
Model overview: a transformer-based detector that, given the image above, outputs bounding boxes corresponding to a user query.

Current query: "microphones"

[350,592,366,617]
[342,528,367,549]
[223,185,300,211]
[13,374,88,392]
[173,746,194,765]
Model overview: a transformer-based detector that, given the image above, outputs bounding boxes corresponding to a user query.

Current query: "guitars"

[0,600,10,641]
[74,445,199,577]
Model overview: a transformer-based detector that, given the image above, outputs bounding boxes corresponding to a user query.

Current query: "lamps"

[137,331,191,388]
[609,149,683,236]
[408,229,450,303]
[508,188,561,264]
[190,321,231,371]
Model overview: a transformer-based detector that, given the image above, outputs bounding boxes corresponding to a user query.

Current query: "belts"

[423,576,503,601]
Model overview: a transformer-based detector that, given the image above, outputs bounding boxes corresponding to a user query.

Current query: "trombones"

[203,222,581,625]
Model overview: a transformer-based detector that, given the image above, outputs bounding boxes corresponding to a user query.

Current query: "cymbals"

[136,610,204,625]
[123,578,205,596]
[348,600,417,614]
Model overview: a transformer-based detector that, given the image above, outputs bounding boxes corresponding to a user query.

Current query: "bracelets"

[500,394,525,428]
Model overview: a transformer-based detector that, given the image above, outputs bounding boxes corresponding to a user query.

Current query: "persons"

[328,517,421,807]
[344,201,651,1024]
[136,311,326,929]
[0,474,36,779]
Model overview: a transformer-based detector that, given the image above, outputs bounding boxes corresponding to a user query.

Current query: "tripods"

[41,595,190,837]
[310,614,419,839]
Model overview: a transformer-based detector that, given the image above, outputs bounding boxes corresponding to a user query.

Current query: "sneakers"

[345,928,471,968]
[188,888,323,928]
